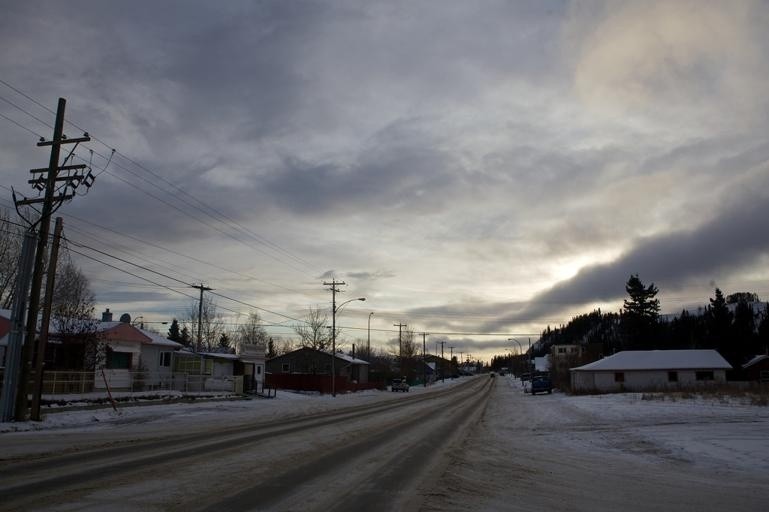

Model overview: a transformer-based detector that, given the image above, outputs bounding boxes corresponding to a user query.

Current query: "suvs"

[390,379,409,392]
[524,376,553,395]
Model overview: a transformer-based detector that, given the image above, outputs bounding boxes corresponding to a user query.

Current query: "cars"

[488,370,505,379]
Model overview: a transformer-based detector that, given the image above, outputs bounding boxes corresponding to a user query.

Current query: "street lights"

[330,295,374,397]
[503,336,525,381]
